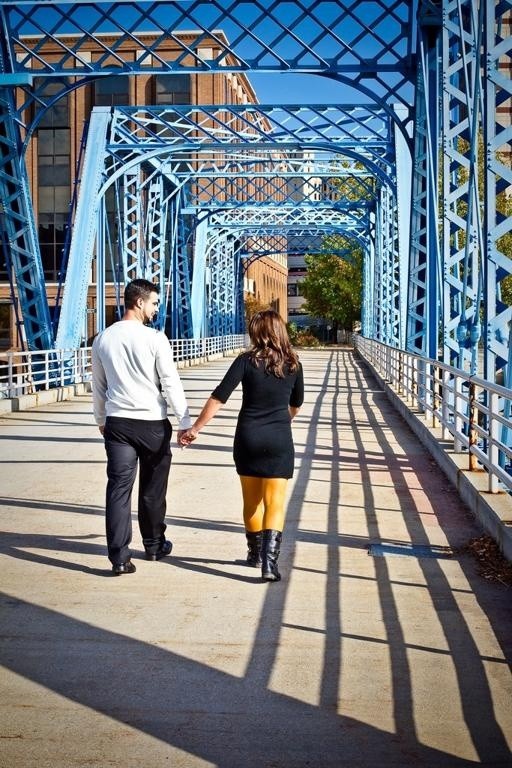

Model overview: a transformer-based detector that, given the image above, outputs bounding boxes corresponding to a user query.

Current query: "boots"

[246,529,282,581]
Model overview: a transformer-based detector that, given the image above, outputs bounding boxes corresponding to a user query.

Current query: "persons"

[91,279,189,576]
[180,311,304,581]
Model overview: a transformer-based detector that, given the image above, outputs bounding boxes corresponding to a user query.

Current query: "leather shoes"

[112,561,136,574]
[145,540,172,561]
